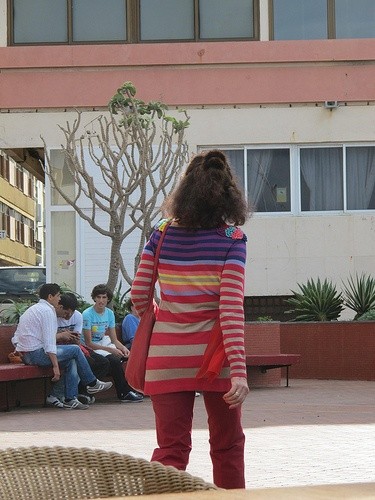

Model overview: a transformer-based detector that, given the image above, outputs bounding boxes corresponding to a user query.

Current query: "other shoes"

[194,392,201,397]
[77,394,95,404]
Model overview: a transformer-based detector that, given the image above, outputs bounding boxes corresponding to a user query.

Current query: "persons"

[131,149,248,490]
[47,295,81,407]
[122,298,141,349]
[58,292,112,404]
[80,284,144,402]
[11,283,113,409]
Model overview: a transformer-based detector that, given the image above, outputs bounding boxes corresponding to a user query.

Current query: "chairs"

[0,441,225,500]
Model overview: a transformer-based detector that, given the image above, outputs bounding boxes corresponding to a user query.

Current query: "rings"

[234,392,240,397]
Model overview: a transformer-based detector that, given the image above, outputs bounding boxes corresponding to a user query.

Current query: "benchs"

[0,363,64,408]
[244,352,303,388]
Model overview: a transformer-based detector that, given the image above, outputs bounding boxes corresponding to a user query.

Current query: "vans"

[0,265,46,304]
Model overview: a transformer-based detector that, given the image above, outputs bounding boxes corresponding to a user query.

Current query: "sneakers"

[118,390,143,402]
[46,395,66,409]
[87,379,113,395]
[63,397,89,410]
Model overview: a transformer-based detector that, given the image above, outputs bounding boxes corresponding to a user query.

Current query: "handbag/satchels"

[125,216,174,392]
